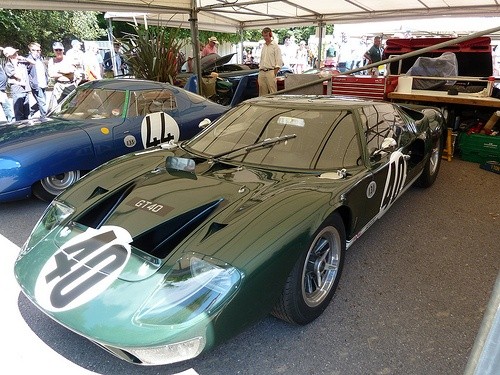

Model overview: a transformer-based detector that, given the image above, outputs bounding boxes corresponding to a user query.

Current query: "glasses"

[55,49,62,51]
[33,48,41,51]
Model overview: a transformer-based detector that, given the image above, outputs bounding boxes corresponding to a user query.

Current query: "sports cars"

[15,95,448,367]
[0,74,250,204]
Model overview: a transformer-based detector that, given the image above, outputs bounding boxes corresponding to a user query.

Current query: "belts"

[260,69,274,72]
[57,81,74,84]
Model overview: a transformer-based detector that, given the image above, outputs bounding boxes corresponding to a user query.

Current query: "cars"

[183,52,355,106]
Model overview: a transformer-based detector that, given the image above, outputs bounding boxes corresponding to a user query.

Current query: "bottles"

[485,76,495,96]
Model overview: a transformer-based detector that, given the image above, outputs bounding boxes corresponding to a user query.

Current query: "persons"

[257,27,282,98]
[103,38,125,76]
[199,36,220,59]
[0,39,104,123]
[120,55,125,74]
[157,40,178,76]
[242,35,386,78]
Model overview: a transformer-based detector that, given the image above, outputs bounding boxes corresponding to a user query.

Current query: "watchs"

[369,58,372,61]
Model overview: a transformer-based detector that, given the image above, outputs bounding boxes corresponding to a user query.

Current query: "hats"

[71,40,82,44]
[3,47,19,57]
[208,37,219,44]
[53,42,64,49]
